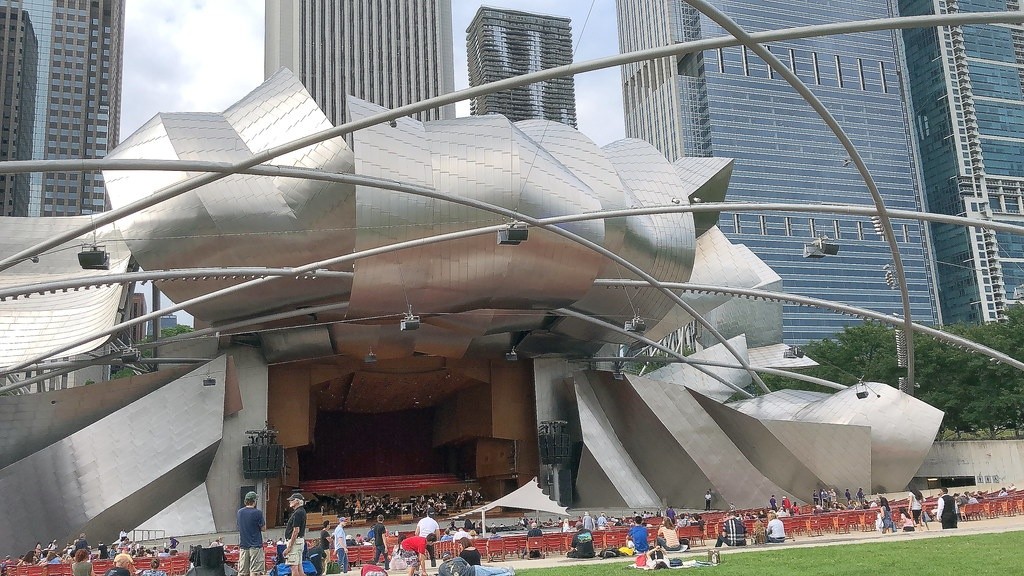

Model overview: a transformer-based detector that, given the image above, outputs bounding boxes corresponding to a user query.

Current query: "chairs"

[0,490,1024,576]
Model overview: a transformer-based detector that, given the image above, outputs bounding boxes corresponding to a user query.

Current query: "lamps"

[363,349,378,362]
[803,239,840,260]
[202,376,217,387]
[121,348,143,363]
[783,347,805,359]
[506,350,517,361]
[623,318,650,332]
[495,220,528,247]
[78,242,111,270]
[399,316,421,330]
[856,391,870,399]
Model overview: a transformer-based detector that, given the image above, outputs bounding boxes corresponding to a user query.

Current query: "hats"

[442,551,451,558]
[531,522,537,529]
[584,511,590,515]
[339,517,345,522]
[287,493,305,501]
[575,521,583,530]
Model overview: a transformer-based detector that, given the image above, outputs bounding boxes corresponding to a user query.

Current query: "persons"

[715,511,747,547]
[770,494,799,517]
[519,511,634,559]
[998,483,1017,497]
[283,493,307,576]
[880,484,925,534]
[626,516,649,554]
[666,506,701,531]
[566,522,595,558]
[632,511,655,518]
[320,508,481,576]
[728,503,766,519]
[954,491,983,522]
[344,488,483,522]
[654,518,680,551]
[236,492,266,576]
[812,488,882,514]
[937,487,958,529]
[704,491,711,511]
[0,529,238,576]
[765,514,785,543]
[267,537,283,546]
